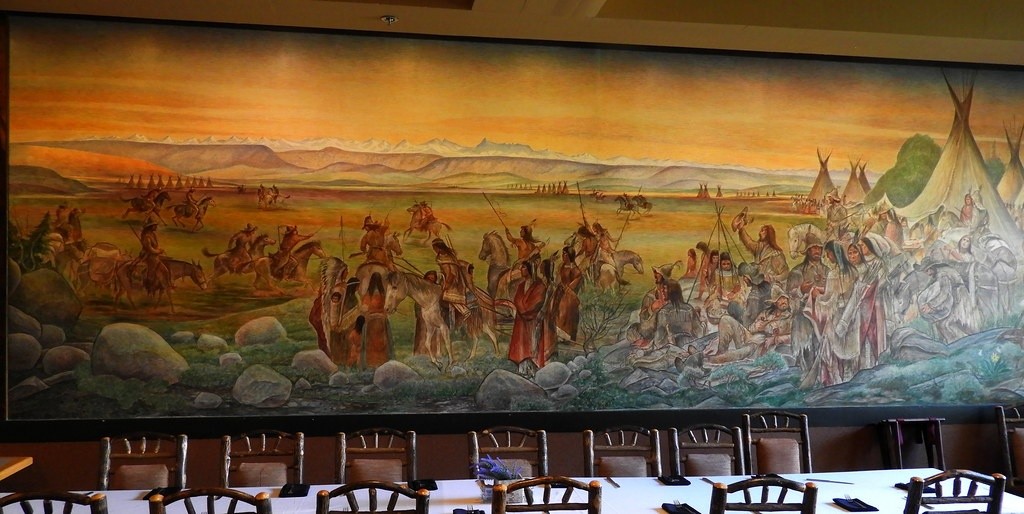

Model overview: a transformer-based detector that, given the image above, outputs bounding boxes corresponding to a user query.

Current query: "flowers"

[469,454,523,482]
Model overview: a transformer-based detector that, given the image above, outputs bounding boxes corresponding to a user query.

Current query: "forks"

[673,499,695,514]
[844,494,866,509]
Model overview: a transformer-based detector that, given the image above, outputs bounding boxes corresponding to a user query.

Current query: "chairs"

[149,486,272,514]
[668,424,746,477]
[335,427,416,484]
[740,410,813,476]
[903,470,1006,514]
[316,480,430,514]
[467,426,548,481]
[0,489,109,514]
[710,477,818,514]
[221,429,305,488]
[996,403,1024,498]
[490,475,602,514]
[101,432,188,492]
[582,425,662,478]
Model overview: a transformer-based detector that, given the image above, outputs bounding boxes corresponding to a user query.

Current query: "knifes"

[607,476,620,488]
[806,479,853,484]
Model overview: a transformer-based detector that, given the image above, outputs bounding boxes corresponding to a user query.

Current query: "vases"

[477,476,525,504]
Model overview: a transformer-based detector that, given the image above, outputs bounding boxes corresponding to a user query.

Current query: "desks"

[0,468,1024,514]
